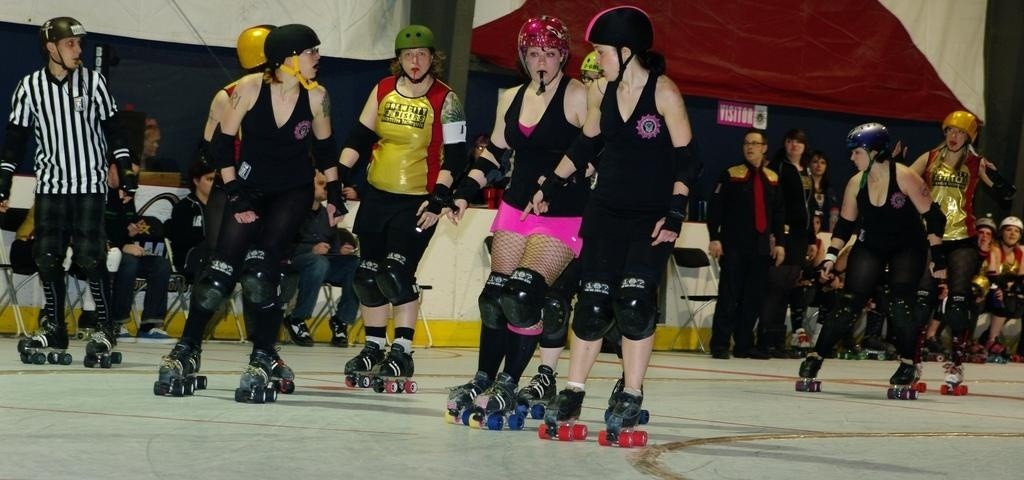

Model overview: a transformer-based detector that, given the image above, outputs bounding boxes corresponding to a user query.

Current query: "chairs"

[732,344,772,359]
[329,315,348,347]
[0,207,433,350]
[672,248,716,355]
[111,324,136,343]
[710,347,729,359]
[136,326,178,344]
[283,314,314,346]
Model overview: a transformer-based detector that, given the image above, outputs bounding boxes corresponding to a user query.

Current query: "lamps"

[672,248,716,355]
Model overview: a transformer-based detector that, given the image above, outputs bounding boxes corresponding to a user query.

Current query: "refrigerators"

[911,362,927,392]
[795,353,824,392]
[84,323,121,368]
[235,342,277,403]
[76,310,98,340]
[33,309,67,336]
[605,372,649,424]
[967,341,988,363]
[462,372,529,430]
[887,357,927,401]
[923,337,950,361]
[371,343,418,393]
[445,371,494,425]
[940,363,968,395]
[18,322,71,365]
[836,336,867,360]
[344,341,386,387]
[599,378,649,448]
[861,333,896,361]
[982,336,1008,363]
[786,328,810,356]
[154,338,207,396]
[1012,342,1023,362]
[271,345,295,394]
[539,389,587,441]
[517,364,558,419]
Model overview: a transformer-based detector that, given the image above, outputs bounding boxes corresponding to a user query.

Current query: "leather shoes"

[661,193,688,238]
[423,183,449,214]
[326,179,349,218]
[119,170,138,198]
[530,171,568,204]
[992,182,1016,198]
[222,179,257,216]
[818,246,839,274]
[930,243,947,273]
[453,175,480,208]
[0,190,11,205]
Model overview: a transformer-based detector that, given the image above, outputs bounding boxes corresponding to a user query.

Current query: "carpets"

[754,173,768,232]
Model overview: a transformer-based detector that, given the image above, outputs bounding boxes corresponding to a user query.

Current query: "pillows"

[237,25,276,68]
[518,15,569,49]
[972,275,991,297]
[999,216,1024,231]
[845,123,893,151]
[586,6,653,51]
[976,218,997,232]
[395,24,435,50]
[264,24,320,57]
[42,17,86,44]
[943,110,978,143]
[580,51,603,74]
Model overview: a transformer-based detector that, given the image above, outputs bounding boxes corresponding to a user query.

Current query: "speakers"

[757,324,784,358]
[776,327,799,358]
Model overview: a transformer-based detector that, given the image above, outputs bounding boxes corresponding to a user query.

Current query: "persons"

[338,25,466,394]
[172,157,217,278]
[538,8,697,445]
[1,17,138,368]
[790,210,1024,364]
[796,123,947,400]
[706,132,832,359]
[909,111,997,395]
[191,25,295,394]
[447,17,587,430]
[142,113,160,158]
[515,53,649,425]
[154,25,349,402]
[11,161,178,343]
[467,135,492,204]
[283,169,359,347]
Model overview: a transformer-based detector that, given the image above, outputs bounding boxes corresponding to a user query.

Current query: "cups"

[744,143,764,146]
[304,47,318,55]
[944,129,966,136]
[580,75,600,83]
